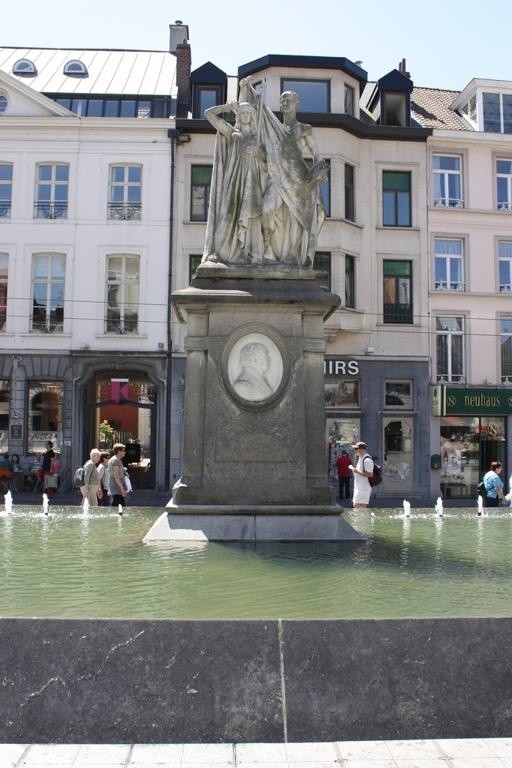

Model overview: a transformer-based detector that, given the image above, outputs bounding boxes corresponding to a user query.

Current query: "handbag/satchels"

[43,474,58,488]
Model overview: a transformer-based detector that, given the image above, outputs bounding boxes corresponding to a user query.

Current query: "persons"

[504,476,512,507]
[0,438,134,506]
[347,441,375,508]
[239,75,321,263]
[335,450,352,500]
[232,343,273,402]
[203,101,267,263]
[482,461,505,507]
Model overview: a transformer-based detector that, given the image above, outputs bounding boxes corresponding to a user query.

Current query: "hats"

[351,441,368,449]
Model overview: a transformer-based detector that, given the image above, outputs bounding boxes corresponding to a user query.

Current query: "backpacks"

[73,466,85,488]
[362,456,382,487]
[475,481,488,497]
